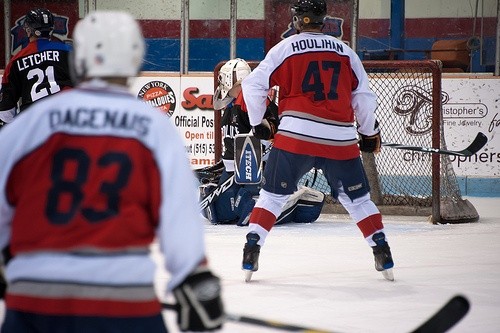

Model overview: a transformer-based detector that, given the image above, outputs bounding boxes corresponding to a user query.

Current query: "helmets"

[24,8,55,37]
[288,0,328,27]
[72,9,145,79]
[217,57,253,100]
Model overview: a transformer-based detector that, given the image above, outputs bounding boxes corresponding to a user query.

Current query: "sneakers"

[241,242,260,282]
[371,242,395,280]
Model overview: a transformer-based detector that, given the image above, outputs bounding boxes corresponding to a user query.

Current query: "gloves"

[252,123,271,140]
[171,270,225,333]
[358,130,382,154]
[0,247,13,299]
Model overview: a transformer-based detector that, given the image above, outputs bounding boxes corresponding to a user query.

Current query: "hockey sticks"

[161,294,470,333]
[253,127,488,157]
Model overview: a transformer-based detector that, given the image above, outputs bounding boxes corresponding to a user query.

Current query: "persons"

[241,0,396,283]
[218,58,280,224]
[0,7,76,122]
[0,11,227,332]
